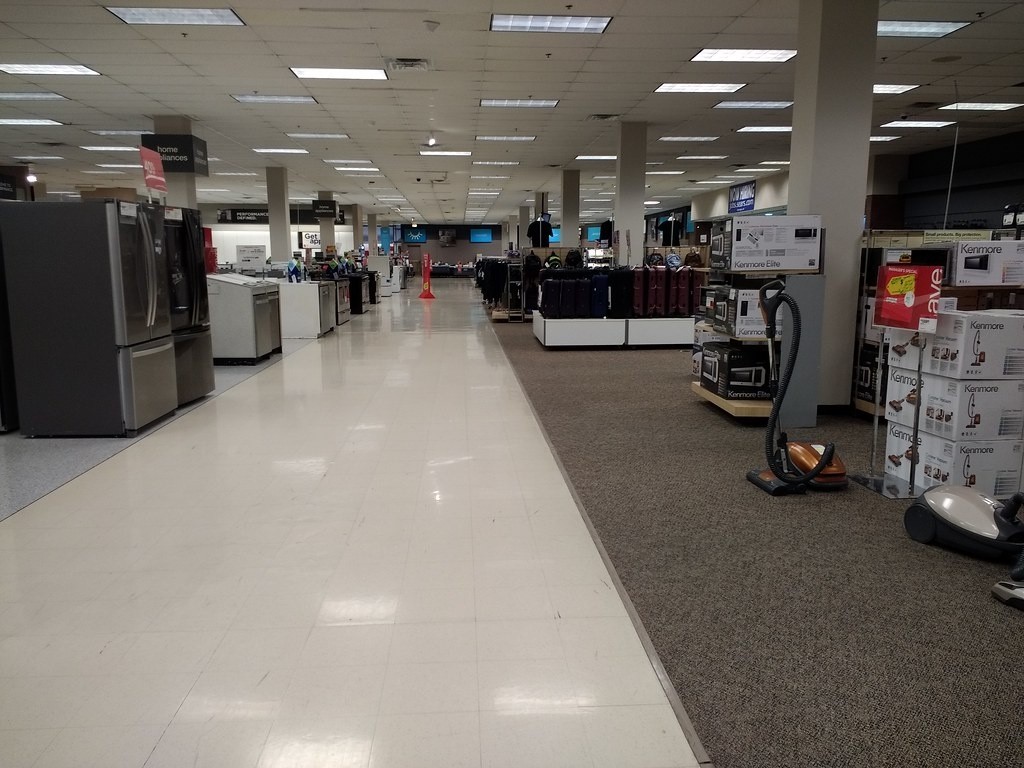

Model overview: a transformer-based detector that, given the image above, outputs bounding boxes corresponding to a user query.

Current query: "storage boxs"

[700,341,780,402]
[882,236,1023,504]
[710,287,783,341]
[708,214,824,277]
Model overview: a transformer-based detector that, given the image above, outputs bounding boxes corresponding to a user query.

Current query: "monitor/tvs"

[549,227,560,243]
[587,226,601,242]
[658,216,670,226]
[686,211,694,232]
[404,228,426,243]
[469,228,493,243]
